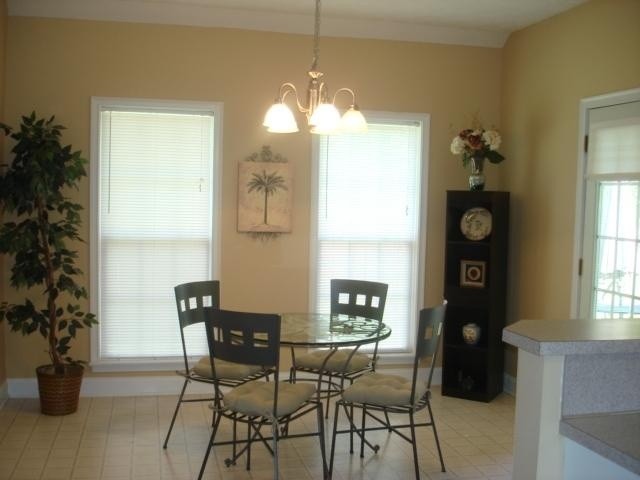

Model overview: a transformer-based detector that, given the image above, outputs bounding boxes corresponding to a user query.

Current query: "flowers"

[449,127,507,168]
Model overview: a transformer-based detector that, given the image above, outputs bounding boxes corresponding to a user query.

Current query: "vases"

[467,156,487,190]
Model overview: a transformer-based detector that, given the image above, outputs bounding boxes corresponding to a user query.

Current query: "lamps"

[261,0,371,138]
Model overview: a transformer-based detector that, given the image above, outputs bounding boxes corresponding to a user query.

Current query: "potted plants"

[0,107,100,416]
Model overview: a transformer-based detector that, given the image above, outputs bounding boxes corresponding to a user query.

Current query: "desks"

[225,310,391,480]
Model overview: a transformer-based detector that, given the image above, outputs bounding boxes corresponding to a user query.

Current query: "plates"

[460,207,493,241]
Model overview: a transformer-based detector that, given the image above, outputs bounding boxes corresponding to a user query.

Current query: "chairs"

[328,301,448,480]
[195,307,329,480]
[282,277,393,435]
[161,279,275,452]
